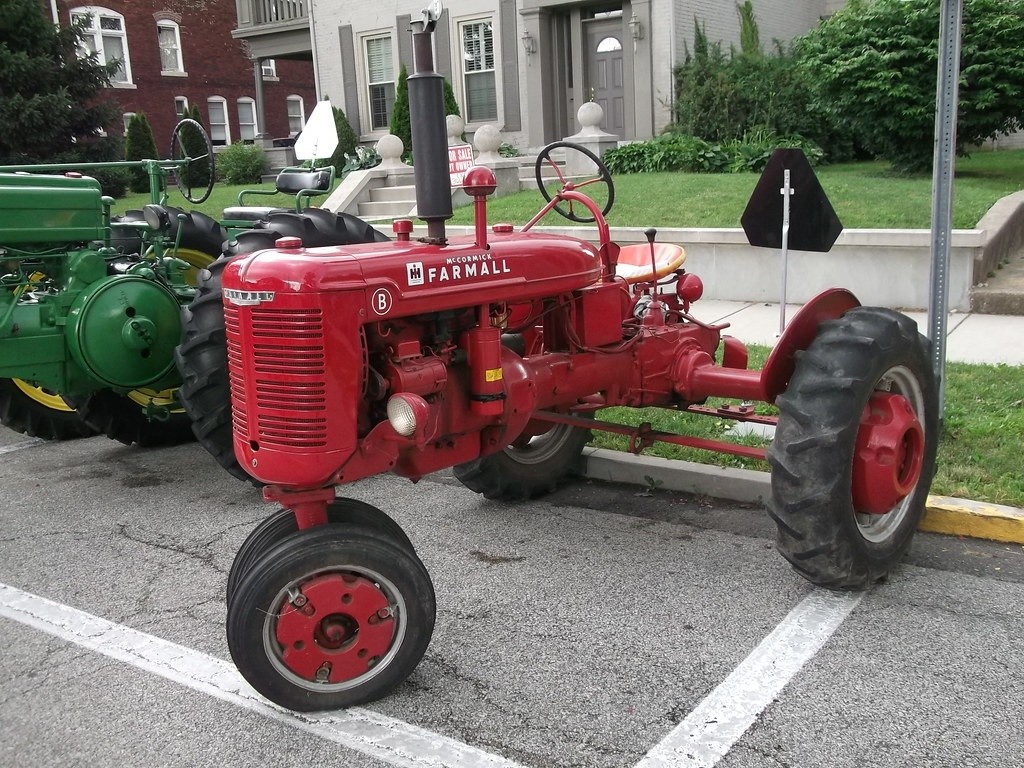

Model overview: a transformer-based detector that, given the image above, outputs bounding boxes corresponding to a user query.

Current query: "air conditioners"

[262,66,273,76]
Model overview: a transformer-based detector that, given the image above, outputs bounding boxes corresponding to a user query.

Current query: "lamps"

[521,28,537,67]
[628,12,644,55]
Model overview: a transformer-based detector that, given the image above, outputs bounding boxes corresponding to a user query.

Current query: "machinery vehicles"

[221,8,943,713]
[0,118,395,486]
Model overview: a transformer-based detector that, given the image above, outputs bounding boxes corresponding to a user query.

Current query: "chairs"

[221,165,336,239]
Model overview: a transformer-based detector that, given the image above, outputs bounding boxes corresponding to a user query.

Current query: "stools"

[612,242,687,285]
